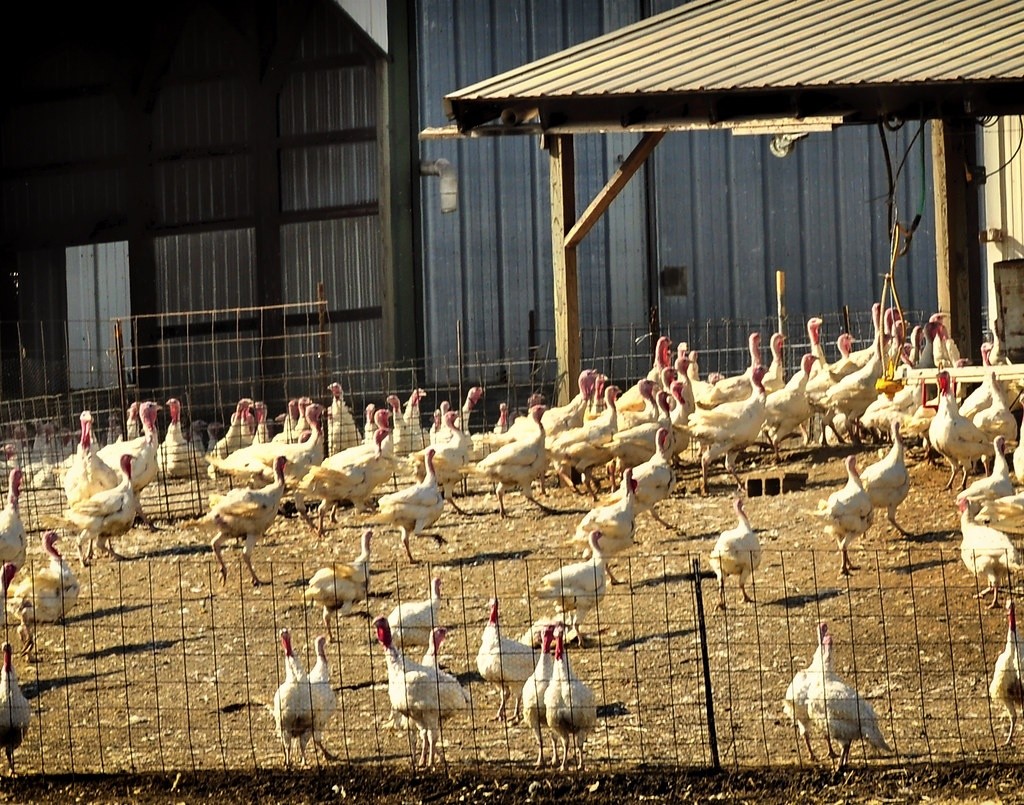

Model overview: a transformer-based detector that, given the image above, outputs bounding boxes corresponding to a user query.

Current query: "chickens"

[0,301,1024,777]
